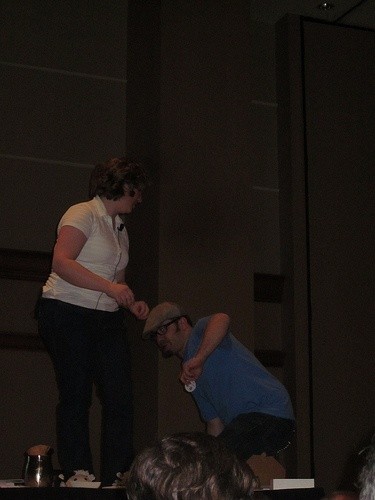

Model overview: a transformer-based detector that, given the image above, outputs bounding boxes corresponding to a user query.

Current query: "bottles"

[24,455,52,488]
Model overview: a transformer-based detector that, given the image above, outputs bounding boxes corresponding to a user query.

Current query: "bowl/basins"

[27,445,48,455]
[70,480,101,488]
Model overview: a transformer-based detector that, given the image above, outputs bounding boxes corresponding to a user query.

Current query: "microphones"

[118,223,124,231]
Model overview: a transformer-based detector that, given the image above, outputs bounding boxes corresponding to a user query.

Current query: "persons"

[142,302,293,488]
[126,431,255,500]
[34,156,153,488]
[356,448,375,500]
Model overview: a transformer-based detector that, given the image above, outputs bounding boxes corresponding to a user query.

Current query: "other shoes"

[54,469,104,488]
[102,471,129,488]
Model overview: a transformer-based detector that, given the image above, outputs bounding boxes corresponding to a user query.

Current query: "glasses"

[149,317,180,341]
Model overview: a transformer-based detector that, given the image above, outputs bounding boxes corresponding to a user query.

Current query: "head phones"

[107,169,137,201]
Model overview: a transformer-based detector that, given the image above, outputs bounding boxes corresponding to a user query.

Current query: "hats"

[142,302,195,340]
